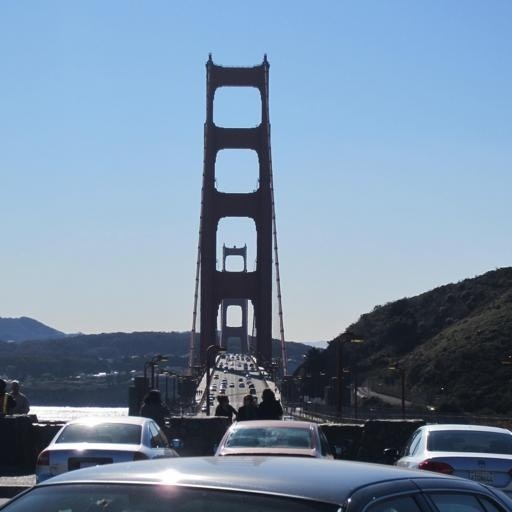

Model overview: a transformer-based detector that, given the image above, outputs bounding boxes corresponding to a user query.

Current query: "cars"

[36,415,181,483]
[164,414,232,455]
[1,456,512,512]
[199,351,267,416]
[215,419,336,459]
[319,419,427,465]
[383,423,512,498]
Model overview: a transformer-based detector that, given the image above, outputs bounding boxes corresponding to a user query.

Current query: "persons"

[140,388,172,432]
[257,389,283,420]
[249,394,258,406]
[234,394,258,421]
[8,379,30,414]
[215,395,238,425]
[0,378,18,415]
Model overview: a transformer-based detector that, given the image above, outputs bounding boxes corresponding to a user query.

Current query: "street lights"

[142,353,195,412]
[284,336,407,420]
